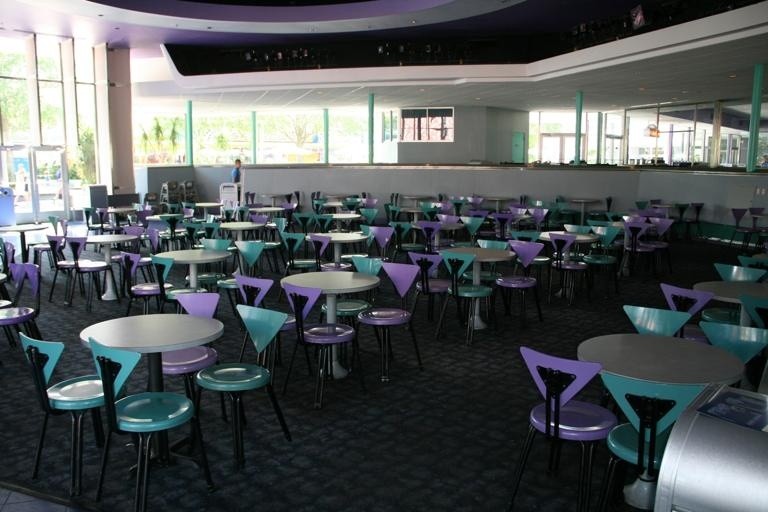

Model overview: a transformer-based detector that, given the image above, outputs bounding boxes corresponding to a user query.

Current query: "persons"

[761,155,768,168]
[56,164,70,199]
[231,159,241,205]
[43,172,51,187]
[13,163,31,207]
[165,154,187,163]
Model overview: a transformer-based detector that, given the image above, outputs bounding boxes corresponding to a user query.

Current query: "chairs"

[467,197,484,209]
[264,217,288,273]
[183,221,202,249]
[674,202,689,233]
[359,208,379,226]
[0,242,16,286]
[358,262,425,374]
[753,212,768,249]
[217,240,265,316]
[133,209,154,233]
[417,221,443,254]
[218,182,240,221]
[279,231,317,278]
[431,250,494,345]
[0,265,49,370]
[250,214,268,240]
[0,262,25,336]
[87,337,218,512]
[173,218,188,232]
[693,321,768,366]
[620,303,692,336]
[597,368,707,510]
[142,192,159,210]
[158,215,183,253]
[160,288,220,441]
[368,226,394,262]
[314,214,334,233]
[65,236,109,308]
[0,241,17,270]
[84,207,104,234]
[335,211,356,231]
[686,201,704,236]
[701,261,767,322]
[292,211,316,235]
[463,239,509,311]
[236,205,249,221]
[361,197,378,208]
[563,223,590,282]
[185,238,232,291]
[492,213,512,242]
[738,293,768,328]
[389,221,425,262]
[317,255,388,360]
[194,303,293,469]
[124,258,174,316]
[738,256,768,269]
[510,229,554,294]
[659,280,716,338]
[509,207,527,229]
[511,345,616,507]
[462,210,497,246]
[640,218,674,270]
[436,213,460,248]
[583,226,619,296]
[527,207,550,231]
[120,252,141,313]
[233,274,287,364]
[621,214,645,224]
[172,208,195,249]
[34,218,68,268]
[341,225,373,263]
[111,225,145,298]
[750,207,764,232]
[547,233,586,297]
[120,227,163,282]
[451,216,485,248]
[96,211,123,235]
[727,206,755,250]
[278,282,355,411]
[16,330,104,498]
[45,234,85,303]
[122,225,145,252]
[201,222,227,274]
[637,200,648,210]
[589,196,613,220]
[496,240,545,334]
[26,215,59,258]
[309,234,353,271]
[449,199,468,216]
[408,250,450,326]
[651,199,661,208]
[588,220,620,226]
